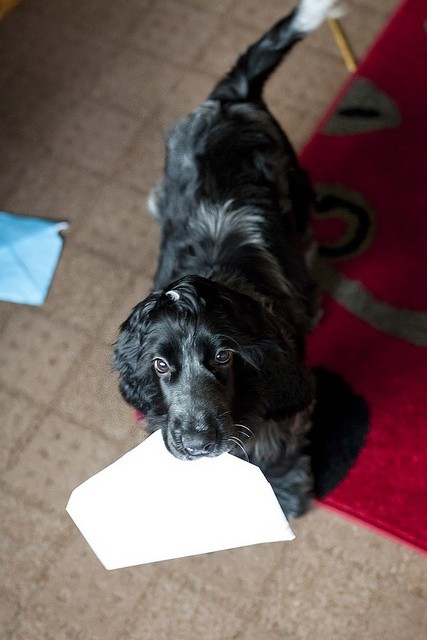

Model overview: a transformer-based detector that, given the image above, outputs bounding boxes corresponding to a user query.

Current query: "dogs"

[113,0,335,524]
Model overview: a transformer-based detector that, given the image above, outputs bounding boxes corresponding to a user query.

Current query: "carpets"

[135,1,426,560]
